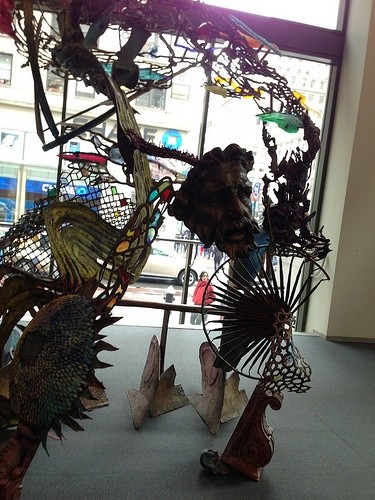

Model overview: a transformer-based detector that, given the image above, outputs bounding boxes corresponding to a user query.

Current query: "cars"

[97,241,199,287]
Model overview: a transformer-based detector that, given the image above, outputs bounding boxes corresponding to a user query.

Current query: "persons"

[272,256,277,273]
[173,231,222,274]
[190,271,215,325]
